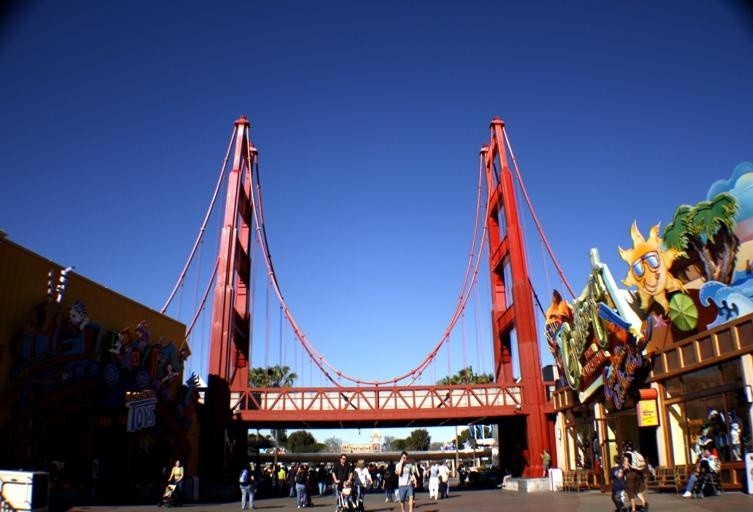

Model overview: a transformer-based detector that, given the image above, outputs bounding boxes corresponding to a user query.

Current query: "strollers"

[157,481,179,508]
[331,479,363,511]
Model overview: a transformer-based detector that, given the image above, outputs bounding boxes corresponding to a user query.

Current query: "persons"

[609,455,628,511]
[238,450,473,511]
[698,408,743,462]
[163,486,173,498]
[643,456,657,483]
[167,460,184,505]
[540,448,552,477]
[681,447,722,498]
[620,440,649,511]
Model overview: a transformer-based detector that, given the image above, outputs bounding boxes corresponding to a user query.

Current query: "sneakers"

[640,502,649,512]
[682,491,693,496]
[302,504,307,507]
[297,505,302,508]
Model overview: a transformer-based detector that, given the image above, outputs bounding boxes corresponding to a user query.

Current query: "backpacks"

[625,450,646,470]
[239,469,248,485]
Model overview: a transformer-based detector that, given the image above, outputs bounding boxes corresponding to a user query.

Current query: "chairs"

[556,463,721,496]
